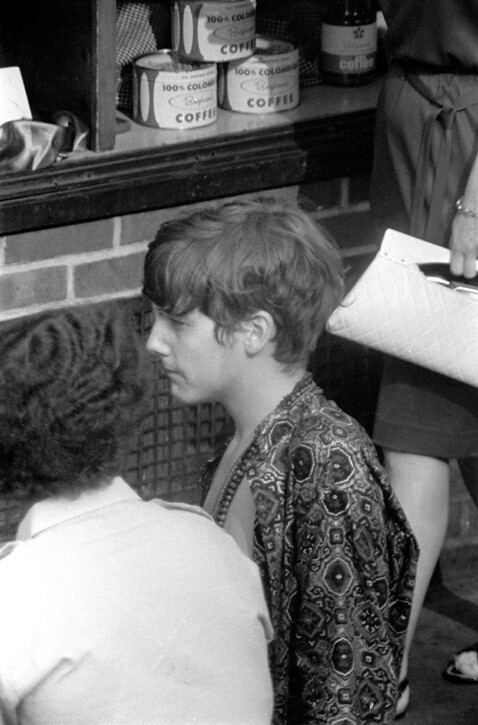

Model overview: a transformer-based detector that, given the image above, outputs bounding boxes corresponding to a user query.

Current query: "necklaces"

[200,382,323,529]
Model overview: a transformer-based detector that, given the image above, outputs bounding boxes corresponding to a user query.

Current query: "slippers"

[394,678,411,721]
[444,642,478,685]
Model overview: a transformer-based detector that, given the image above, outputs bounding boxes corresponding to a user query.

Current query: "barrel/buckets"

[131,0,301,130]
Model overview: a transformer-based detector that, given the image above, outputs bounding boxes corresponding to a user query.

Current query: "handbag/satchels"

[324,228,478,386]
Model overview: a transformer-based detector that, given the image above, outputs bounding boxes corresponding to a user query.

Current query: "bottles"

[317,0,379,87]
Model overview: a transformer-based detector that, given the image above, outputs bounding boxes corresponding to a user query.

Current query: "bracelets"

[456,196,478,220]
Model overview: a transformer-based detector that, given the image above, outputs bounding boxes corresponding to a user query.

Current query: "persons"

[0,302,279,725]
[376,0,478,717]
[141,193,420,725]
